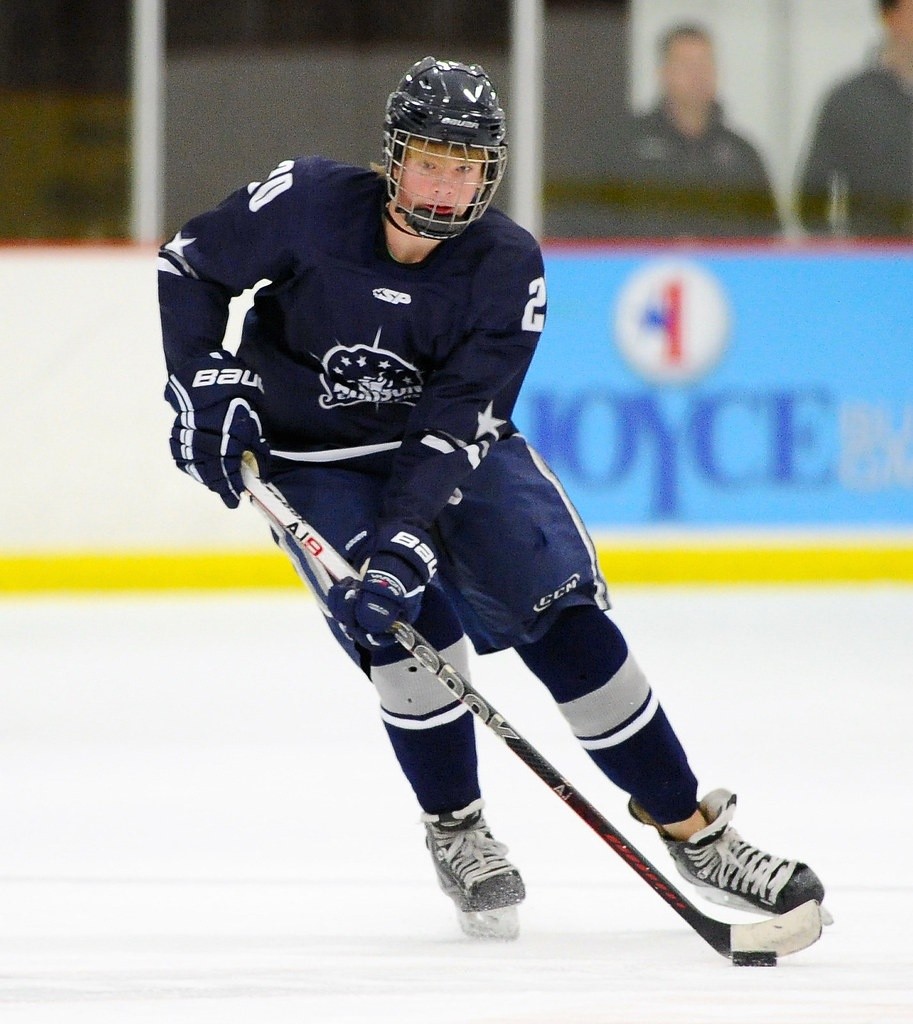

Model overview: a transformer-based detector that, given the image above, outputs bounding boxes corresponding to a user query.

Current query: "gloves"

[164,352,269,509]
[326,519,439,652]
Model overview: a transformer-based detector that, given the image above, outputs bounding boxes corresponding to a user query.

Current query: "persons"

[156,58,836,939]
[603,22,785,237]
[796,1,912,239]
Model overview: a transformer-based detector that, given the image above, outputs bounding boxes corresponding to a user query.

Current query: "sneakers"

[423,796,530,940]
[626,782,831,929]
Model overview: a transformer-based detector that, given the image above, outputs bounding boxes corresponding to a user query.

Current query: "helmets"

[382,55,509,242]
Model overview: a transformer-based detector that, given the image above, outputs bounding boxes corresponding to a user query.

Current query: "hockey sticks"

[234,459,830,969]
